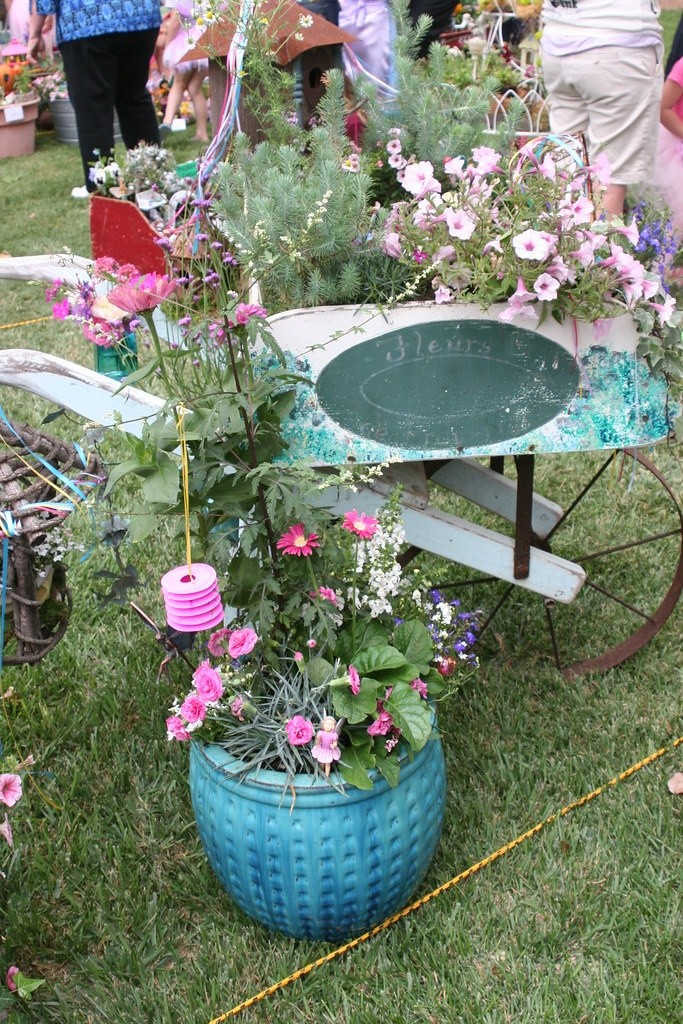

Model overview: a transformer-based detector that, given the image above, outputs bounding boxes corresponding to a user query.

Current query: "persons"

[158,0,683,222]
[26,0,164,198]
[4,0,54,62]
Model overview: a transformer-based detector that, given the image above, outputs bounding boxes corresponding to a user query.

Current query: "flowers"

[0,63,69,105]
[45,0,682,813]
[451,3,472,31]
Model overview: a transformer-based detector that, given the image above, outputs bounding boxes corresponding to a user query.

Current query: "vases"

[440,30,471,48]
[47,99,122,143]
[0,96,41,158]
[188,694,448,939]
[247,263,681,467]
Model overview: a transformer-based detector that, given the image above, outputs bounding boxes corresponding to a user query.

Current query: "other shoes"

[159,124,172,141]
[71,186,90,198]
